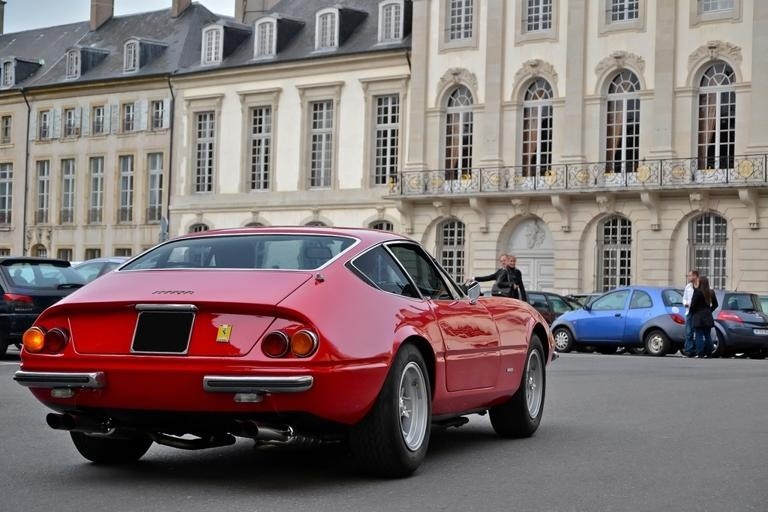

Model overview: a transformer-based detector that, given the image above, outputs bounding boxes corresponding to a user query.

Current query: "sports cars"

[16,227,554,476]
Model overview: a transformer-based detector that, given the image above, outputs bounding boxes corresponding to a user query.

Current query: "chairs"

[215,239,333,269]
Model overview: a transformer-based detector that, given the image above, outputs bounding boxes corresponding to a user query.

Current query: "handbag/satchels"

[491,282,515,297]
[691,309,714,329]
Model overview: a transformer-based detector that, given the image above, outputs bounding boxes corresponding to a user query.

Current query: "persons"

[682,270,700,358]
[465,254,510,284]
[688,276,718,358]
[497,255,527,302]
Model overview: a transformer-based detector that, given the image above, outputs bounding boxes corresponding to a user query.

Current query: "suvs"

[0,254,88,363]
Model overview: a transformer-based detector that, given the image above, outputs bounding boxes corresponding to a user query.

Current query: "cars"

[69,257,137,283]
[479,280,768,359]
